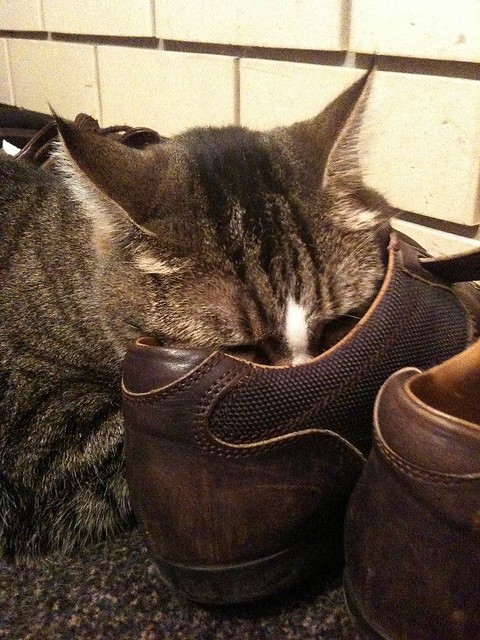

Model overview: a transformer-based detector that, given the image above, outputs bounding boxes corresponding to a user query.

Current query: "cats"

[0,51,399,555]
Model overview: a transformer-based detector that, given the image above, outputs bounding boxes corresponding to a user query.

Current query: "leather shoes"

[344,334,480,636]
[121,232,480,610]
